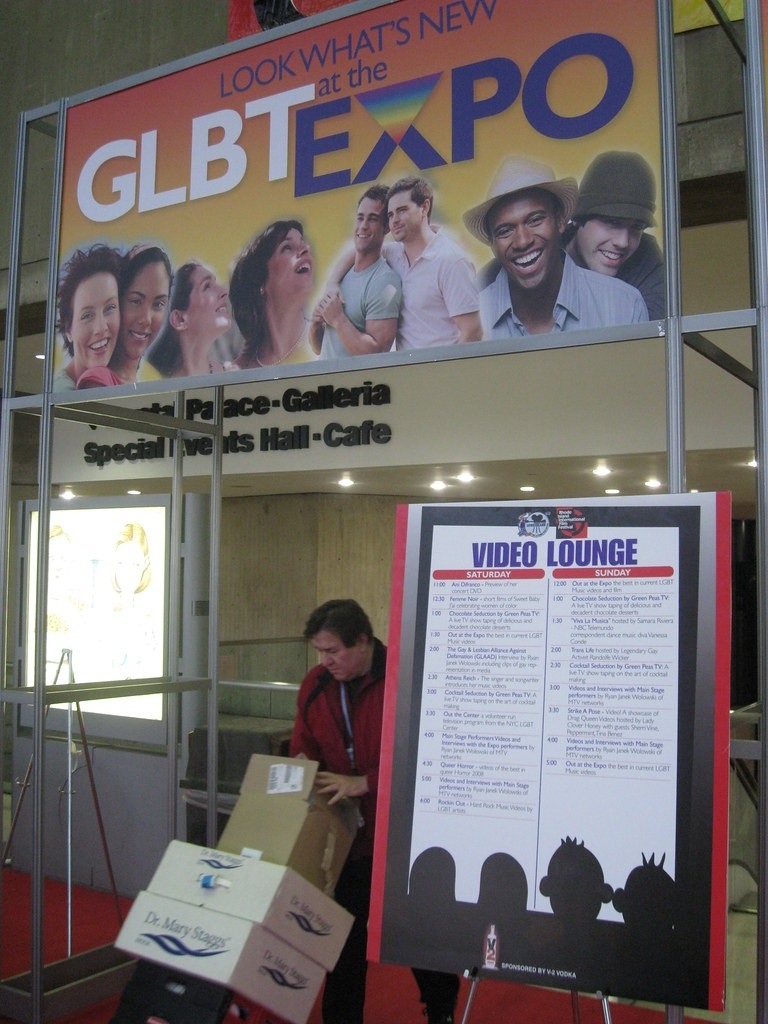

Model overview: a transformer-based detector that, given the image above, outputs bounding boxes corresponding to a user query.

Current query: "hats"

[462,155,579,246]
[570,151,657,227]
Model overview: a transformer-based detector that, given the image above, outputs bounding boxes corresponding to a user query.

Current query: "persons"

[323,175,484,353]
[146,262,240,379]
[76,244,173,391]
[290,599,461,1024]
[229,220,322,370]
[477,151,668,319]
[461,156,649,343]
[307,185,401,360]
[52,245,121,395]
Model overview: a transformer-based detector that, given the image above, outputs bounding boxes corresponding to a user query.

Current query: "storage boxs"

[114,890,327,1024]
[148,839,356,972]
[217,752,362,896]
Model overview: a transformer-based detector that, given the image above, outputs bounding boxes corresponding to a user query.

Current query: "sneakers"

[427,1012,455,1024]
